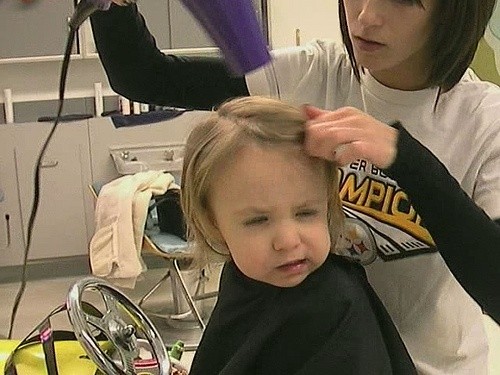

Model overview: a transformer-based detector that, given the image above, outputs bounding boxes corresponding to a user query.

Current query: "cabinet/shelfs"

[0,0,79,64]
[80,0,269,60]
[1,123,93,270]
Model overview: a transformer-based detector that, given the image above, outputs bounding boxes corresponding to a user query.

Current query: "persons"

[180,97,418,375]
[85,0,500,375]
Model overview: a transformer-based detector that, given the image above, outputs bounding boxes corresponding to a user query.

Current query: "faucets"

[165,149,173,160]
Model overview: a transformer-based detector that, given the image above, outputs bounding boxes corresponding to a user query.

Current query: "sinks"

[110,146,184,175]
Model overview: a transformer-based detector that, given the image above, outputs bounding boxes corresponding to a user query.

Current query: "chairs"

[90,171,219,351]
